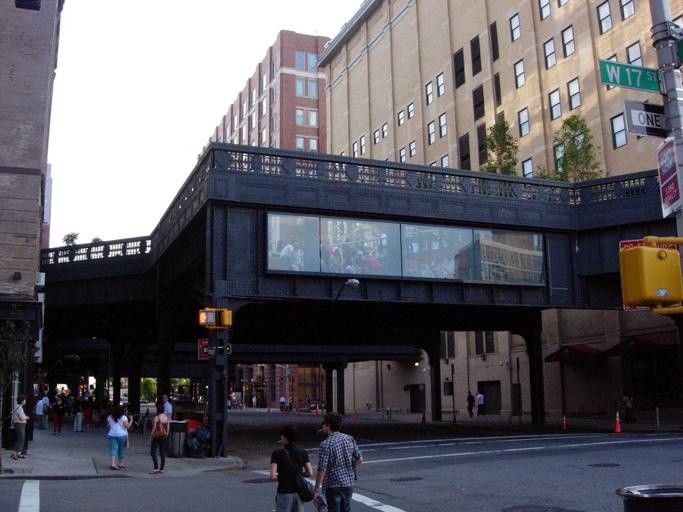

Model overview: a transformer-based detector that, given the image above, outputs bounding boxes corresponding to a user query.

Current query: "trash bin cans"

[167,421,189,457]
[615,483,683,512]
[280,402,285,412]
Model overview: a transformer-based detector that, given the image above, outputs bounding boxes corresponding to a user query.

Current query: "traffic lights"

[199,309,214,325]
[220,310,232,327]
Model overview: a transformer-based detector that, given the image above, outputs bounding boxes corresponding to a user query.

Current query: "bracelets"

[315,486,320,493]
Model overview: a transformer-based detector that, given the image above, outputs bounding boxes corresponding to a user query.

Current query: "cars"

[75,385,147,405]
[154,391,243,410]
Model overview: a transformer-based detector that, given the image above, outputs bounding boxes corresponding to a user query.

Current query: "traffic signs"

[599,57,661,94]
[656,137,682,218]
[624,97,668,138]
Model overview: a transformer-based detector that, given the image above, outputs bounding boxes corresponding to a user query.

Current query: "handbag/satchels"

[295,475,315,501]
[153,422,166,437]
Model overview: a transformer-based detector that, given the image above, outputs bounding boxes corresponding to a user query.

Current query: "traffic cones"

[450,414,457,427]
[506,416,514,431]
[266,405,320,416]
[559,415,569,431]
[612,412,621,433]
[419,410,427,424]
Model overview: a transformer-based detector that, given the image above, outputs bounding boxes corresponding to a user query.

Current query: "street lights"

[275,363,289,405]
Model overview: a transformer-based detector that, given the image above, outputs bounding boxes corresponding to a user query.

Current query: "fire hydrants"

[386,407,392,419]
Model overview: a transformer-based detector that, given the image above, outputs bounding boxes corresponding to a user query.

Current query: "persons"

[269,427,313,512]
[149,405,170,472]
[621,390,633,424]
[270,227,465,275]
[104,404,134,470]
[465,390,474,419]
[251,394,257,410]
[314,409,363,512]
[160,393,172,426]
[278,395,284,411]
[11,389,111,459]
[475,391,485,416]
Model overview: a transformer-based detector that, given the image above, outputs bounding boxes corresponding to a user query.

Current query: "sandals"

[10,454,26,460]
